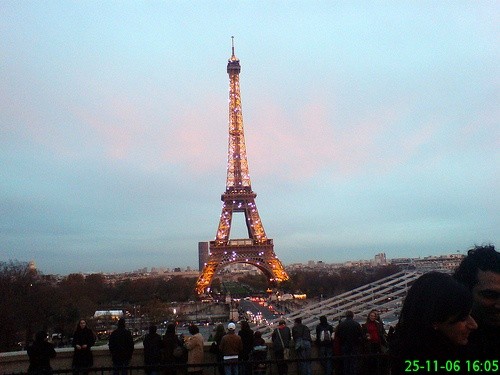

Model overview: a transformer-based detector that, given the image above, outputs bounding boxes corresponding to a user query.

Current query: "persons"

[72,319,95,375]
[386,244,500,375]
[27,331,57,375]
[109,318,134,375]
[143,309,389,375]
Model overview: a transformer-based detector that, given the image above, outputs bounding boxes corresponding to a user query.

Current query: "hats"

[227,322,236,331]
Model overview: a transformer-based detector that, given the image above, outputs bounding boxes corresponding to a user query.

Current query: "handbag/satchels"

[283,348,290,360]
[294,337,304,351]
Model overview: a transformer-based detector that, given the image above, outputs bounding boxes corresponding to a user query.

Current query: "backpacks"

[319,324,331,342]
